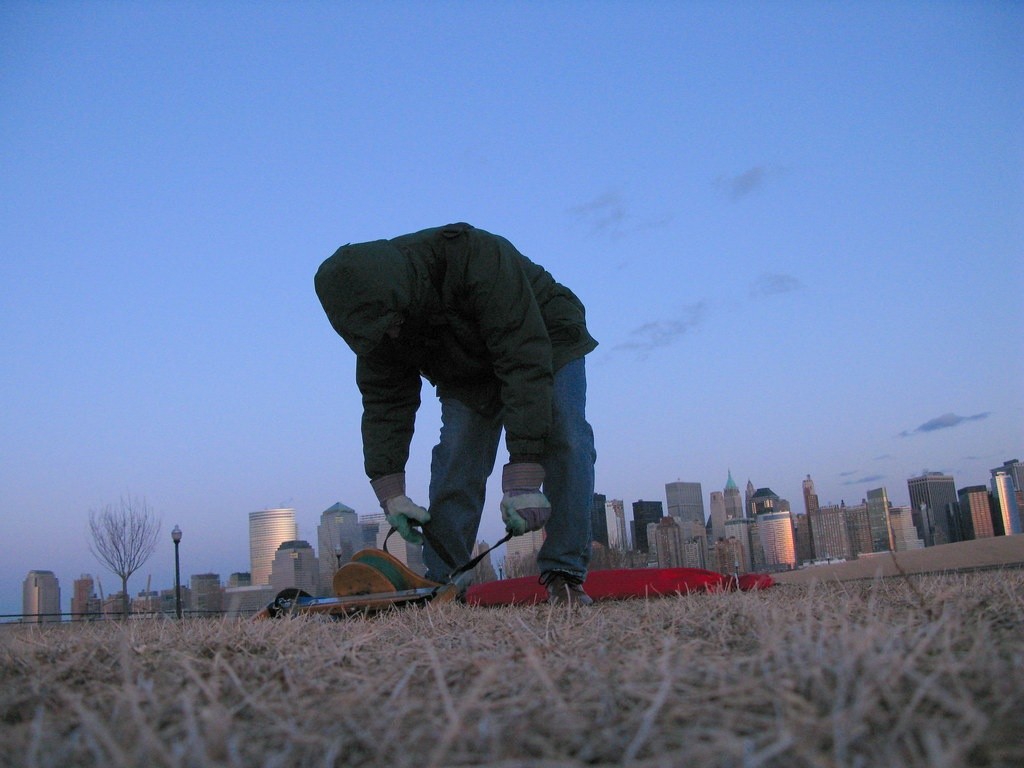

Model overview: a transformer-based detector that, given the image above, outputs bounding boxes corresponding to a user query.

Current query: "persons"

[314,220,600,604]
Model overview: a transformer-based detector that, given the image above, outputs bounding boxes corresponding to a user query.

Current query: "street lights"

[826,552,831,564]
[734,561,741,578]
[170,523,182,620]
[334,543,343,567]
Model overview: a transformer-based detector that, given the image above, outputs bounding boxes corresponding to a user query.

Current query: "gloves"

[368,472,435,547]
[497,460,550,538]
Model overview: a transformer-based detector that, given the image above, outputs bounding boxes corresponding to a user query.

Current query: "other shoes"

[538,569,605,610]
